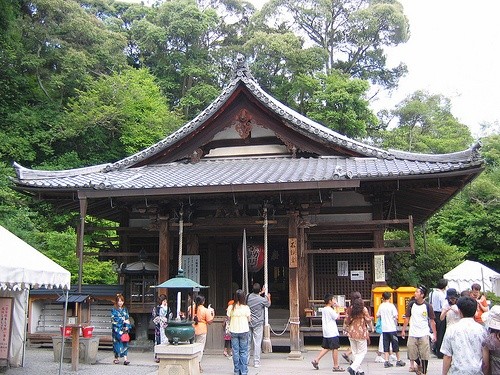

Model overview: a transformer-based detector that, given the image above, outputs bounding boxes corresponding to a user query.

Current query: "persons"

[189,294,215,372]
[244,282,272,368]
[222,314,235,357]
[438,297,487,374]
[430,279,448,358]
[341,290,376,375]
[111,295,131,365]
[439,283,490,328]
[312,294,346,372]
[401,283,437,375]
[152,294,173,363]
[226,290,251,374]
[378,291,406,367]
[480,305,500,374]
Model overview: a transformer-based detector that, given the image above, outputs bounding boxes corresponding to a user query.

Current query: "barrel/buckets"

[81,326,95,339]
[59,326,72,337]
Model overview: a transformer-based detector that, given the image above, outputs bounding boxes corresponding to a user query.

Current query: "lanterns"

[236,238,268,282]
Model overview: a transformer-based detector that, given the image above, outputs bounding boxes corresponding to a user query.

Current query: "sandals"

[342,353,353,365]
[332,366,345,372]
[311,359,319,370]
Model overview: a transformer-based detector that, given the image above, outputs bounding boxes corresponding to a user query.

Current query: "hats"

[419,284,427,291]
[228,300,234,305]
[481,305,500,330]
[445,288,459,299]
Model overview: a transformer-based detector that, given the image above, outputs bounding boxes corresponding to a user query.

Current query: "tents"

[443,259,500,297]
[0,226,71,374]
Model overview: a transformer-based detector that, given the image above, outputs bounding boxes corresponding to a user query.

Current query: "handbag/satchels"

[375,317,382,333]
[121,327,130,341]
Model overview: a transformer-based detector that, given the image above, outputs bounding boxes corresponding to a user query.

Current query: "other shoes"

[347,367,356,375]
[389,356,397,361]
[416,373,426,375]
[231,352,233,356]
[124,360,130,365]
[254,362,262,368]
[409,367,418,372]
[396,360,406,366]
[375,356,388,362]
[356,371,364,375]
[224,352,229,356]
[384,362,393,367]
[155,358,160,362]
[114,360,120,363]
